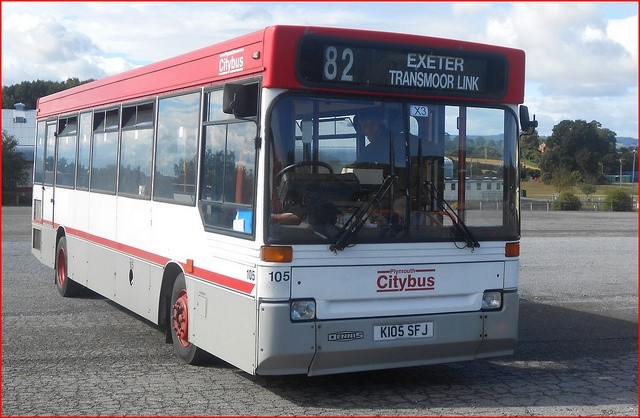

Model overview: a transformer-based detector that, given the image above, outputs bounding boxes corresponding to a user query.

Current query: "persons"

[353,111,443,169]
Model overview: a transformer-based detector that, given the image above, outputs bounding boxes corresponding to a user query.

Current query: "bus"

[32,25,537,377]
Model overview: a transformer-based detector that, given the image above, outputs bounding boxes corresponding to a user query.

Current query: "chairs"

[235,134,305,226]
